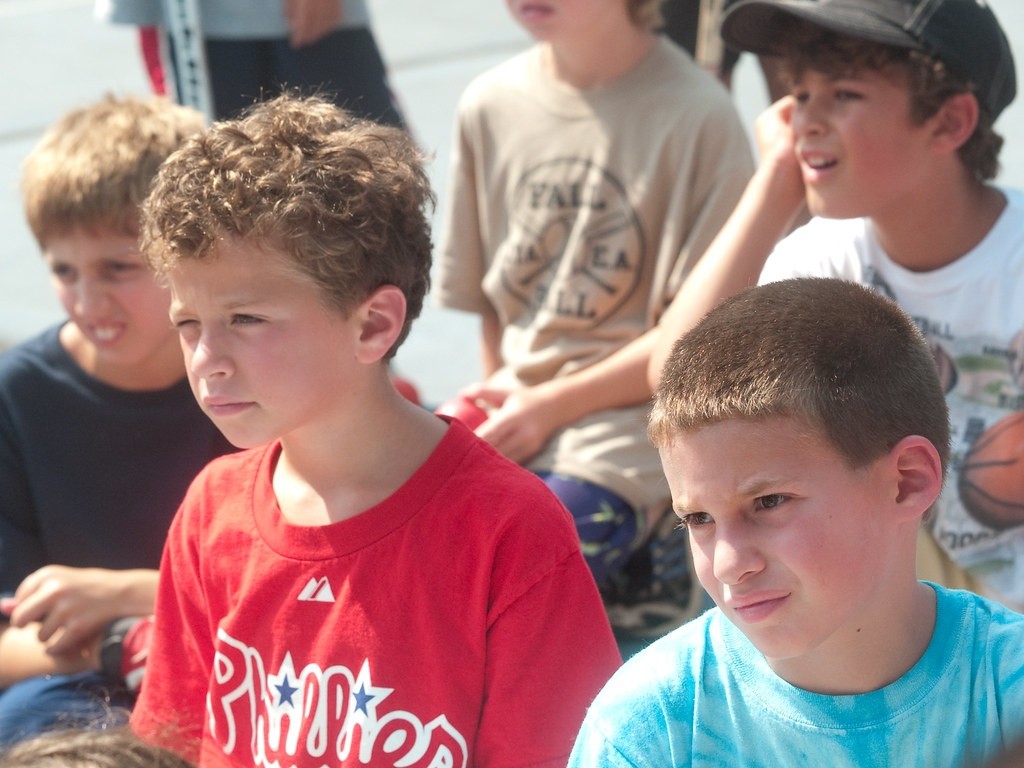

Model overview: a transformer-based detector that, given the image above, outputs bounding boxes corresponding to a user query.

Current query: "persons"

[0,95,243,768]
[126,93,622,768]
[564,278,1023,767]
[100,0,412,138]
[435,0,756,583]
[646,1,1024,613]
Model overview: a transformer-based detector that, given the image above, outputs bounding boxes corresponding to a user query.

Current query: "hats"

[720,0,1016,126]
